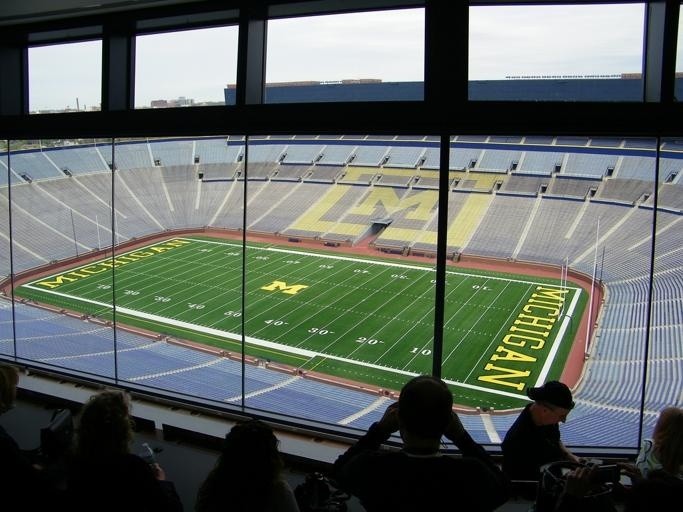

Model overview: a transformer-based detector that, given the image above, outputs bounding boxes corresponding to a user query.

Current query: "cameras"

[596,465,620,485]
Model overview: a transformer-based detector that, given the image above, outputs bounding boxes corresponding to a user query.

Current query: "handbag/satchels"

[291,460,352,511]
[542,460,612,506]
[38,407,74,458]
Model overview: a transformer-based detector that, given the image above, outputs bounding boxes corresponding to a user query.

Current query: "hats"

[400,378,453,433]
[526,381,572,408]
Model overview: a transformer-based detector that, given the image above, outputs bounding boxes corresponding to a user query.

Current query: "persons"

[559,458,681,510]
[328,374,511,510]
[56,388,182,511]
[195,420,297,510]
[0,362,46,511]
[499,380,583,481]
[636,405,683,511]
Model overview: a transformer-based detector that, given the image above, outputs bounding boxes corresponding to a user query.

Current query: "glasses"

[546,406,566,423]
